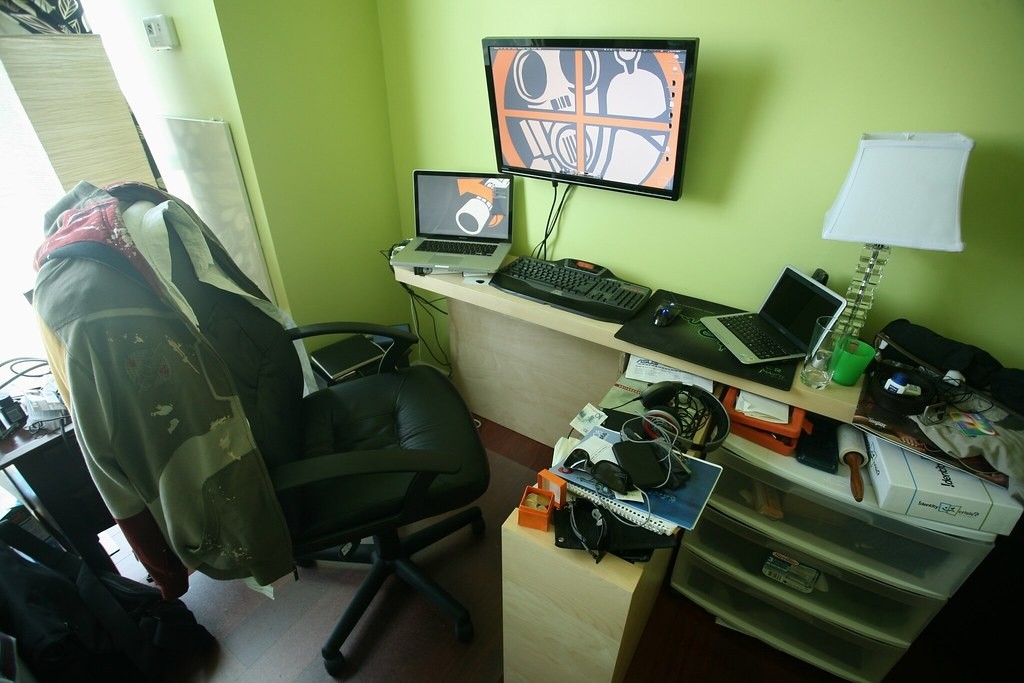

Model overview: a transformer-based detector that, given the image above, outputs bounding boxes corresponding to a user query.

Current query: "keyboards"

[487,256,652,320]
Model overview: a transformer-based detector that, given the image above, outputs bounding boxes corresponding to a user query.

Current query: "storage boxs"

[866,434,1024,536]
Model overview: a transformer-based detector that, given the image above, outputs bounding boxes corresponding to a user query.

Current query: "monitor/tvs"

[482,37,700,200]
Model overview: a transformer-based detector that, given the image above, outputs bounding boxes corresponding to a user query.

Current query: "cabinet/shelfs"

[669,421,996,683]
[0,390,120,578]
[503,383,725,683]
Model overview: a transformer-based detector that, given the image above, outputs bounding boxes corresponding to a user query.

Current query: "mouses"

[652,302,684,327]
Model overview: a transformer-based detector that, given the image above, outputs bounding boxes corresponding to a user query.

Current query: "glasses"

[563,449,631,495]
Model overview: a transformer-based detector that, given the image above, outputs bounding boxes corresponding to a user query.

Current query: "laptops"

[388,171,514,273]
[699,266,847,365]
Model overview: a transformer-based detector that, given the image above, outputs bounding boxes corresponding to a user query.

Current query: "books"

[564,483,681,536]
[549,425,723,536]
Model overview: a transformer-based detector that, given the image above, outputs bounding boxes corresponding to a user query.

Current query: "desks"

[389,252,866,683]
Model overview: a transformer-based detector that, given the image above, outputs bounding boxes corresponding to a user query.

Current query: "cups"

[801,316,854,390]
[829,337,876,386]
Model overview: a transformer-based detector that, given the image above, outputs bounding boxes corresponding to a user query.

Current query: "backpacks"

[0,520,216,683]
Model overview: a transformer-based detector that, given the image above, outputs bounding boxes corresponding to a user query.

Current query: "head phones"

[639,380,731,455]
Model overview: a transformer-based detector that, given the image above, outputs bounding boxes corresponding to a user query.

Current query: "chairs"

[117,197,491,677]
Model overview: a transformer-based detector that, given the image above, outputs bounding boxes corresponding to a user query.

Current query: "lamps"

[821,132,976,345]
